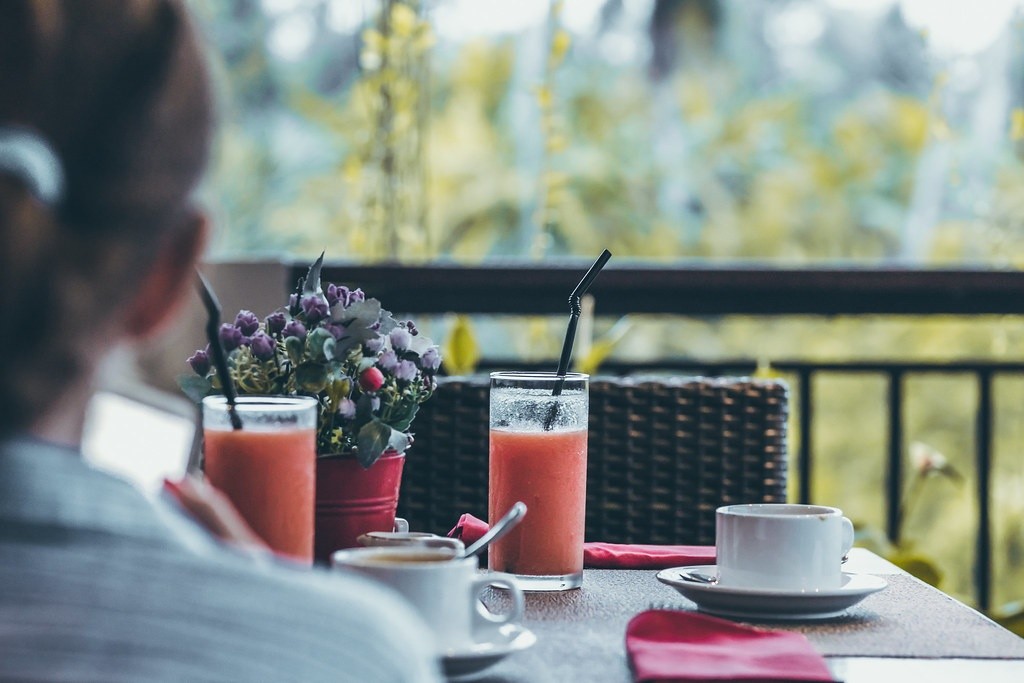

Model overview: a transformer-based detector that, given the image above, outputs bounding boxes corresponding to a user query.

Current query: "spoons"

[679,555,849,582]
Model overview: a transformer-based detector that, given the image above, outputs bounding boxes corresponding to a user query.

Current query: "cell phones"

[79,378,203,506]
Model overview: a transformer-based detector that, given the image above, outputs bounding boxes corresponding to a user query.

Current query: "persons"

[0,0,448,683]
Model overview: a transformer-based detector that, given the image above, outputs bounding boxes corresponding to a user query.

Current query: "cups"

[356,531,466,554]
[329,547,522,648]
[714,504,854,594]
[201,393,315,571]
[487,370,590,591]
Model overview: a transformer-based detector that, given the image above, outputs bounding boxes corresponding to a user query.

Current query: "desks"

[448,539,1023,683]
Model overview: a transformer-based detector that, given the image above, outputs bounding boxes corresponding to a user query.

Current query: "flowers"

[187,284,444,457]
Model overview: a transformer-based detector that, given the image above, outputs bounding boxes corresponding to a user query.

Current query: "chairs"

[372,355,793,534]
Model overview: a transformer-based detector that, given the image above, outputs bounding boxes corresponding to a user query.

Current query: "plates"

[656,564,888,619]
[434,623,537,679]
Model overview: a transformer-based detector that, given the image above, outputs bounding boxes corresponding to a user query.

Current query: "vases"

[314,451,406,571]
